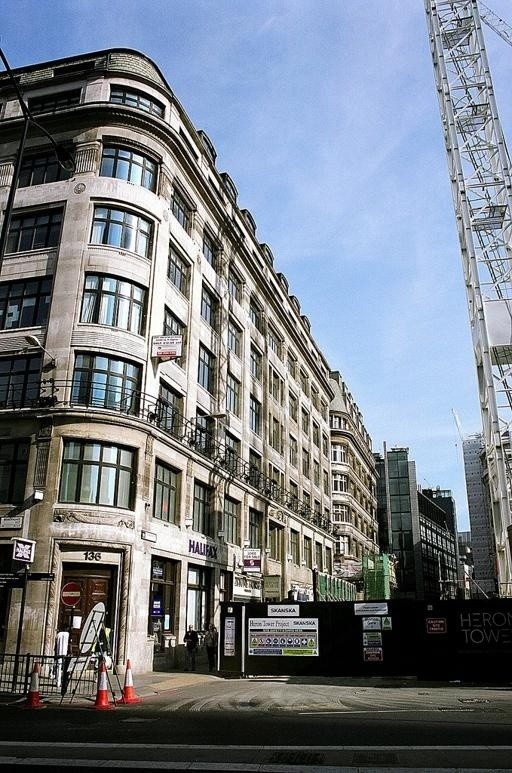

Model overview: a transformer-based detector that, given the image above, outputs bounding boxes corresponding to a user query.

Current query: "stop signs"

[61,582,81,608]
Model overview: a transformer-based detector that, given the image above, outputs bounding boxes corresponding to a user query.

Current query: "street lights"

[0,48,77,278]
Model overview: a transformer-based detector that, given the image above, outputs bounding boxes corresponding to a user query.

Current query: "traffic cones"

[21,662,47,708]
[116,657,141,705]
[88,657,116,710]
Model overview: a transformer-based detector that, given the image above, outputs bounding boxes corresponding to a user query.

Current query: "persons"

[184,625,199,671]
[204,624,218,672]
